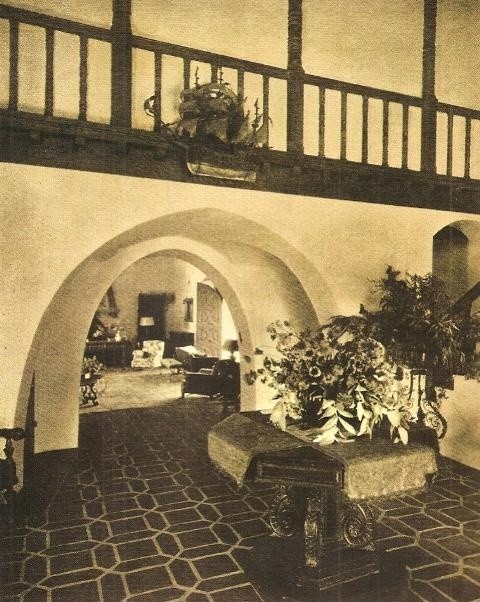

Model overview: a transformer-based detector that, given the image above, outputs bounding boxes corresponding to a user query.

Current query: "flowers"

[244,319,419,444]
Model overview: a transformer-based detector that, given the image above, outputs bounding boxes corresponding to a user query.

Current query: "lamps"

[139,316,154,340]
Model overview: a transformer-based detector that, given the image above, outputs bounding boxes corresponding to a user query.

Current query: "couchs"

[131,340,165,371]
[181,368,216,399]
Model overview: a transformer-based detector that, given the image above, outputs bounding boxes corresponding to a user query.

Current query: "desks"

[170,347,220,375]
[77,374,104,407]
[208,411,436,596]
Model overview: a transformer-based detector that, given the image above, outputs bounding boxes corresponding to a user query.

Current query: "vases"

[301,398,368,426]
